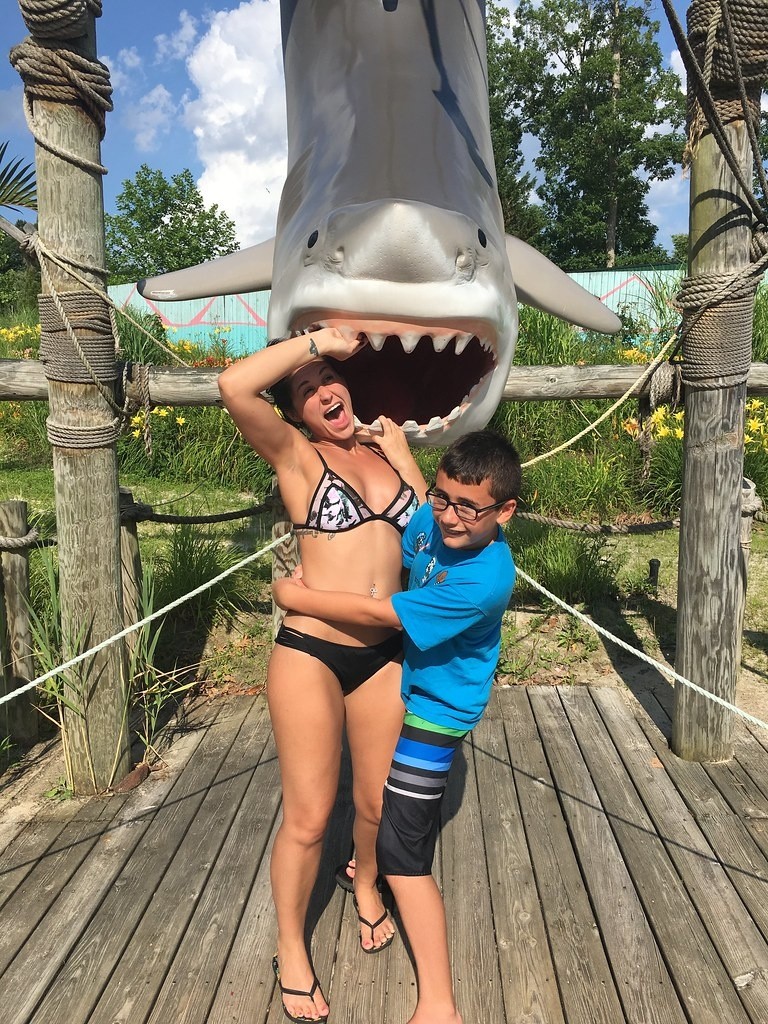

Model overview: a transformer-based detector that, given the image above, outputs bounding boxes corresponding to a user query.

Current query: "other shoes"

[334,862,391,893]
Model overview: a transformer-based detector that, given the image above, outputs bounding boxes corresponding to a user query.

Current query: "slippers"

[271,952,327,1024]
[353,894,394,954]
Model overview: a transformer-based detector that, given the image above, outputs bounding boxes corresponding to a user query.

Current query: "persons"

[214,323,431,1023]
[332,427,525,1024]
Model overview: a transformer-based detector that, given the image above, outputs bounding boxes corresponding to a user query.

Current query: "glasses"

[425,482,506,521]
